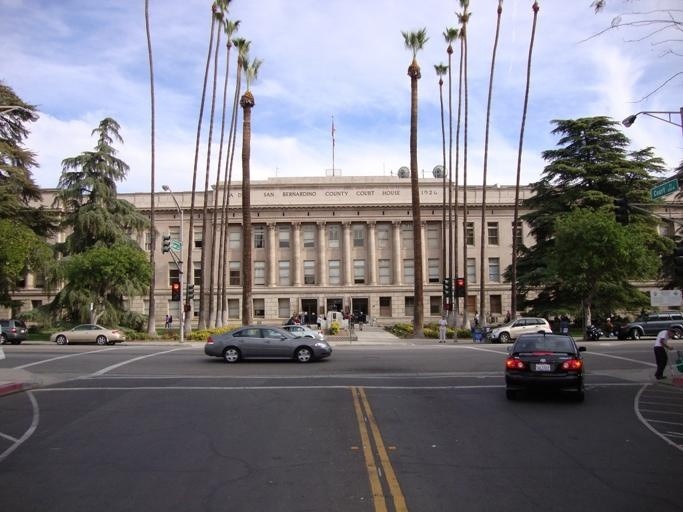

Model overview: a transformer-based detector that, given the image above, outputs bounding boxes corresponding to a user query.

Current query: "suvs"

[617,312,682,342]
[0,319,28,345]
[487,317,552,344]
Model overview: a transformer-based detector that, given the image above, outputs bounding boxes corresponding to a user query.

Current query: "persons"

[654,328,676,380]
[437,310,510,344]
[165,315,172,328]
[285,310,366,332]
[554,307,647,338]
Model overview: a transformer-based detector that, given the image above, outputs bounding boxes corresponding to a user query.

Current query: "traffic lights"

[442,277,452,300]
[454,278,464,299]
[161,232,171,255]
[186,283,194,302]
[171,280,180,302]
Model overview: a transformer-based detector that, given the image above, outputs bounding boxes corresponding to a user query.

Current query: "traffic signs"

[172,240,180,250]
[651,179,678,199]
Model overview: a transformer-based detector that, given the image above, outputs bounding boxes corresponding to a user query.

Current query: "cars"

[270,324,324,341]
[203,324,332,363]
[49,324,125,346]
[505,335,586,401]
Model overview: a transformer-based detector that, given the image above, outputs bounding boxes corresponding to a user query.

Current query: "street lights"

[161,182,184,346]
[620,106,682,133]
[0,104,39,123]
